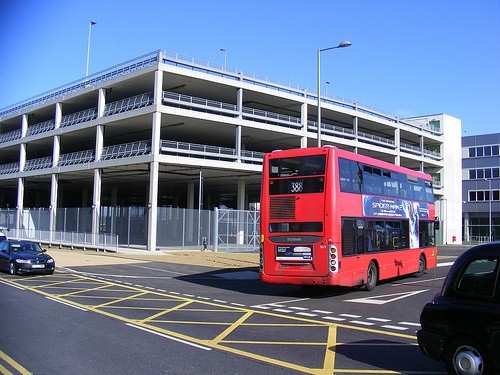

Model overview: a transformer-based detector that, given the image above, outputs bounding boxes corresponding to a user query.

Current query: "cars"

[416,240,500,375]
[0,238,55,274]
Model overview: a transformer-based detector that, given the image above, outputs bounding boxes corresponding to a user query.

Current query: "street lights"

[220,47,227,70]
[85,20,97,87]
[316,41,352,147]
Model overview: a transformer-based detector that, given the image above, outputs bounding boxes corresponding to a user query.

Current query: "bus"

[259,145,440,290]
